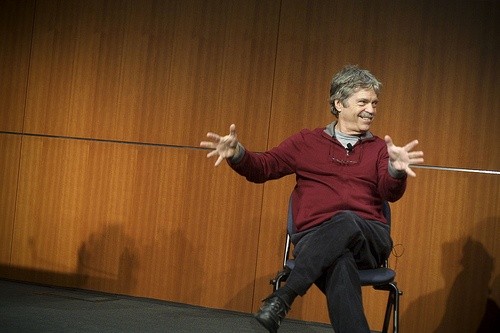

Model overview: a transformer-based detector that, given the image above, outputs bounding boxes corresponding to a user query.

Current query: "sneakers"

[255,288,292,333]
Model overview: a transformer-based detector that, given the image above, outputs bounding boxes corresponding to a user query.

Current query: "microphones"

[346,144,353,151]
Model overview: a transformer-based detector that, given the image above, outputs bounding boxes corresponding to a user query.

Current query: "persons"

[199,63,427,333]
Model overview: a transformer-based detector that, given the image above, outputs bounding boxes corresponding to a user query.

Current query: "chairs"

[269,182,403,333]
